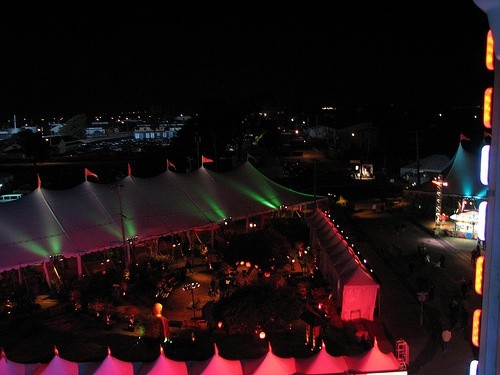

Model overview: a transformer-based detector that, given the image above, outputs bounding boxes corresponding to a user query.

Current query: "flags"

[34,156,214,187]
[0,335,379,359]
[459,134,472,140]
[484,131,491,138]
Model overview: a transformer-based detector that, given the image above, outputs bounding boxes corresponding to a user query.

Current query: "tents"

[350,155,377,181]
[311,204,378,323]
[401,140,488,216]
[0,162,329,290]
[0,345,409,375]
